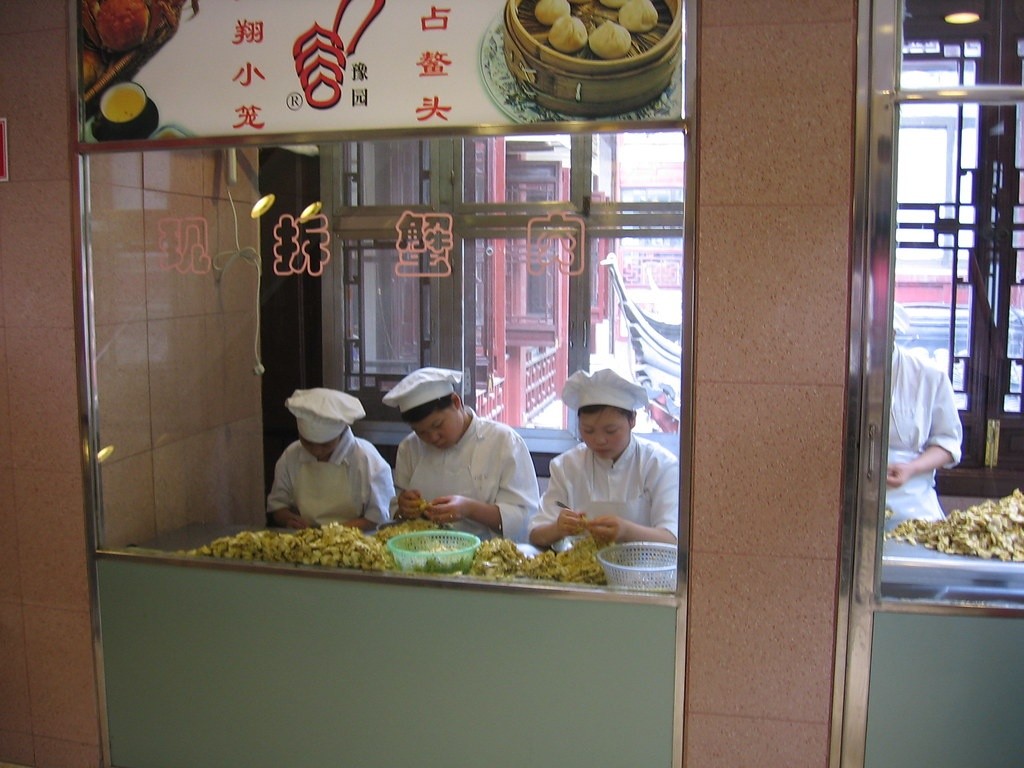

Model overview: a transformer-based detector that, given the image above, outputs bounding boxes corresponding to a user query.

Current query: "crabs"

[79,0,201,58]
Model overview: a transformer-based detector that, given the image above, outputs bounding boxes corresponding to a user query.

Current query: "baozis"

[533,0,658,60]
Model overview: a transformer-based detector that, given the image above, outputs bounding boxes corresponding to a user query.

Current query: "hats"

[561,368,650,411]
[284,388,366,443]
[382,367,463,412]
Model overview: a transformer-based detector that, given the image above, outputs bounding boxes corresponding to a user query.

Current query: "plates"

[92,99,160,142]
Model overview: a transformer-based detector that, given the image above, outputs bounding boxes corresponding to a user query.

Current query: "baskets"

[599,541,676,589]
[386,530,481,576]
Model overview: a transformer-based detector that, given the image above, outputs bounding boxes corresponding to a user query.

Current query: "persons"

[883,329,963,532]
[267,388,397,530]
[382,367,540,541]
[527,369,679,547]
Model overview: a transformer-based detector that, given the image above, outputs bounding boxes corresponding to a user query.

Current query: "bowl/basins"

[551,535,588,555]
[377,522,452,549]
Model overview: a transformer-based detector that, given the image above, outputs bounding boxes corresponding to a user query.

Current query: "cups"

[94,83,150,131]
